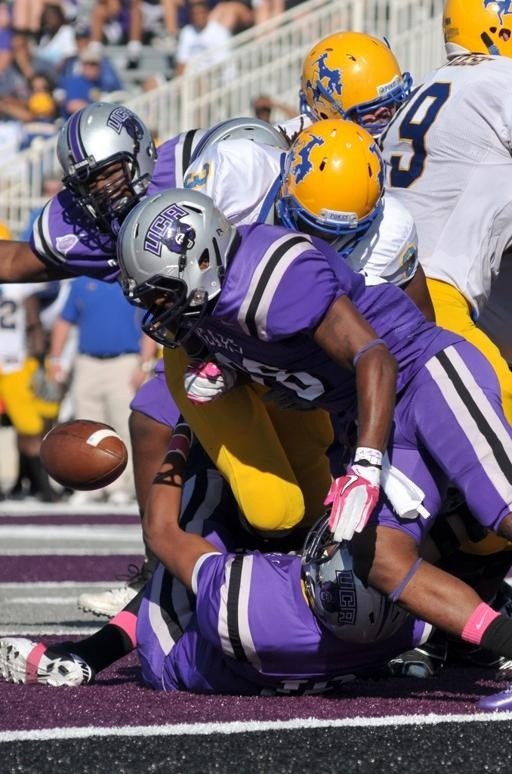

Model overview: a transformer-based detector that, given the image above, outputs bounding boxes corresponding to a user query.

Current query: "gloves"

[183,357,234,404]
[323,450,381,544]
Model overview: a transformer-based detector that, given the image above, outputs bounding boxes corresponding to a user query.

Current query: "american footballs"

[39,419,128,491]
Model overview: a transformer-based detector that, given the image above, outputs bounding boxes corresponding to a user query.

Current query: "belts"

[90,354,120,358]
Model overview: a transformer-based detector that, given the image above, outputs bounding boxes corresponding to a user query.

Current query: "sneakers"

[389,643,462,680]
[1,635,92,691]
[76,562,153,620]
[472,657,512,681]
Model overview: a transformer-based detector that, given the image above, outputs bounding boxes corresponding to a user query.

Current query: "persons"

[0,1,510,713]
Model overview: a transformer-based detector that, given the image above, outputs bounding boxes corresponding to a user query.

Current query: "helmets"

[301,514,405,645]
[443,0,512,59]
[194,117,290,156]
[277,118,386,244]
[119,186,237,351]
[300,31,414,132]
[55,102,157,223]
